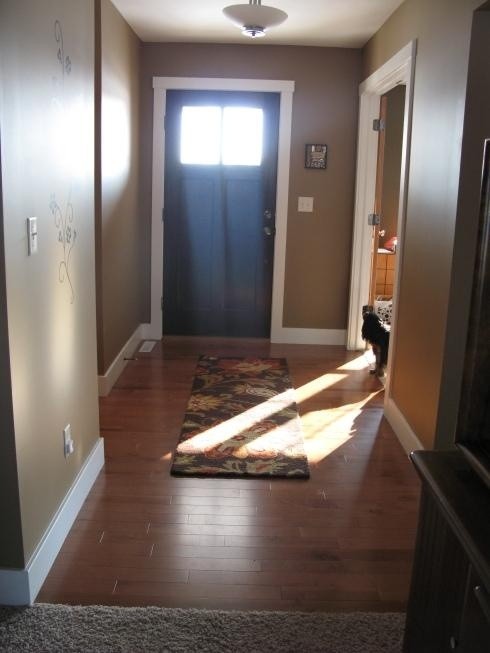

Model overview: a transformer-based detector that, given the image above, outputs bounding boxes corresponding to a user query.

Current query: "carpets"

[171,354,311,478]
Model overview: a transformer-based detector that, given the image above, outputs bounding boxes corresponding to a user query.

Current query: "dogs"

[362,310,391,376]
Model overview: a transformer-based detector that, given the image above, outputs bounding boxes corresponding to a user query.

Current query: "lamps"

[221,1,289,39]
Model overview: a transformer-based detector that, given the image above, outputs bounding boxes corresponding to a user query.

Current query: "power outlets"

[64,424,73,460]
